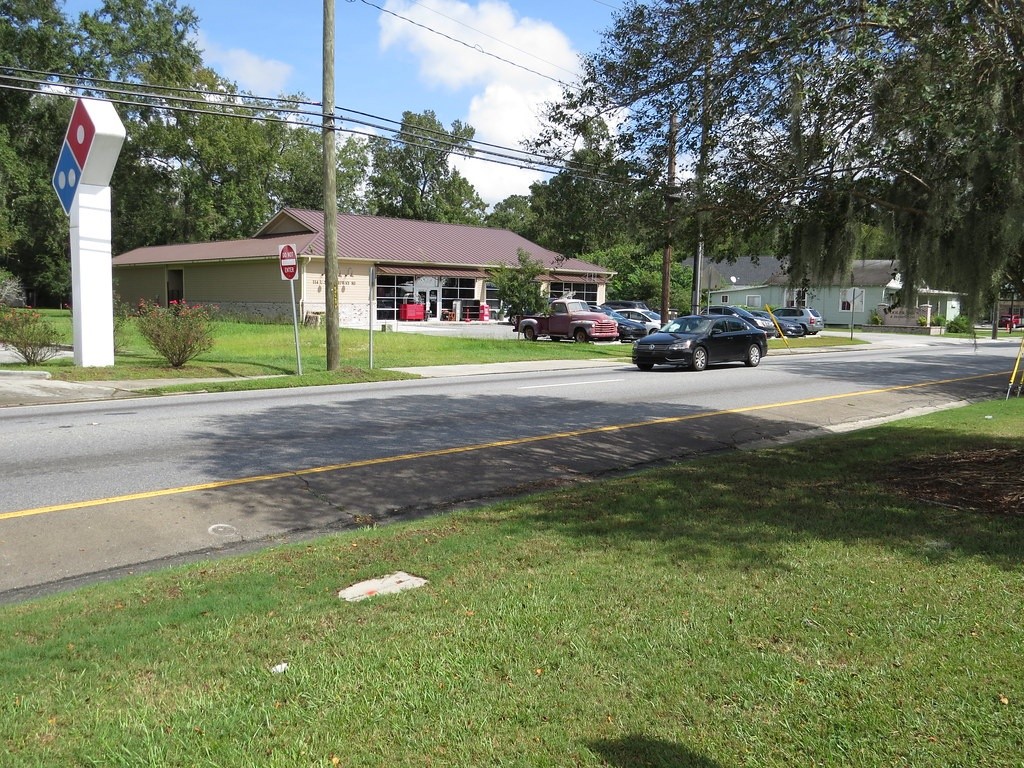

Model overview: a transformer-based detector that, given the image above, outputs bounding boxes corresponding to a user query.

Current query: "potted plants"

[498,308,505,321]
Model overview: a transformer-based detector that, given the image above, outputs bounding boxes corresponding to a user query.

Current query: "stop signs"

[279,244,298,280]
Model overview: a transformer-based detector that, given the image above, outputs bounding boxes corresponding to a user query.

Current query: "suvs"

[699,304,777,339]
[770,306,825,336]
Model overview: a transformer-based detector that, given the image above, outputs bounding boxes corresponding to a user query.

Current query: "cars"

[747,310,805,338]
[600,300,680,322]
[588,305,647,344]
[998,314,1024,329]
[631,313,768,372]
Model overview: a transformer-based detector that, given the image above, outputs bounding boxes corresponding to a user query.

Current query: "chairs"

[690,324,698,331]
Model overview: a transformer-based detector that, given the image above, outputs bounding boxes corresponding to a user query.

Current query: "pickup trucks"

[512,299,620,344]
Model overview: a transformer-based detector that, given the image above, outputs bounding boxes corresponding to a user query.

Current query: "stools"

[448,312,455,321]
